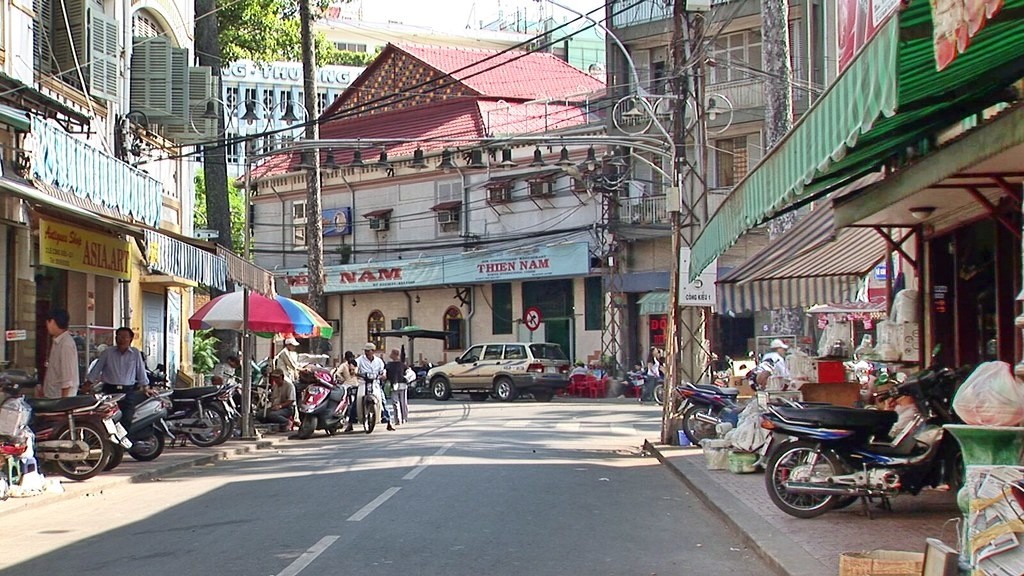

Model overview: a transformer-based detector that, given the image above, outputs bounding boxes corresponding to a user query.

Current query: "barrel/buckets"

[711,440,732,470]
[678,430,694,446]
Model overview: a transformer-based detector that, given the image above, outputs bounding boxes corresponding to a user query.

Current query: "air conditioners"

[529,180,552,194]
[489,189,512,200]
[370,218,389,230]
[391,320,406,330]
[438,209,459,223]
[326,320,337,332]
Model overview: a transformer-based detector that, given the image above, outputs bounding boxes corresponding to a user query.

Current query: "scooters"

[674,356,781,448]
[758,343,976,514]
[653,380,665,406]
[296,367,383,437]
[0,352,244,481]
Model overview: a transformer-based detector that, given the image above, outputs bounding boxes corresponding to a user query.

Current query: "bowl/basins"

[768,391,801,406]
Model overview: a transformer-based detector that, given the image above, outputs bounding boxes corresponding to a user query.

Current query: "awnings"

[688,0,1024,285]
[0,177,145,240]
[714,171,916,288]
[635,291,671,316]
[476,169,562,190]
[804,300,888,329]
[362,209,393,220]
[429,201,462,210]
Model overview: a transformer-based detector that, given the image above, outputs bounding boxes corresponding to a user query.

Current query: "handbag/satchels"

[404,367,416,384]
[952,359,1024,426]
[0,395,32,438]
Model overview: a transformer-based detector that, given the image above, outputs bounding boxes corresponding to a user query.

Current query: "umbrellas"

[187,291,333,439]
[372,325,452,363]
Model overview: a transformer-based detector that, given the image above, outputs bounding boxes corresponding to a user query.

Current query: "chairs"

[569,374,608,398]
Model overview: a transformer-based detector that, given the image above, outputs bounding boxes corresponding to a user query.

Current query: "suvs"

[425,341,572,401]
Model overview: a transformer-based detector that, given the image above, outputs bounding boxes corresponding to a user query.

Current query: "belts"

[106,383,135,390]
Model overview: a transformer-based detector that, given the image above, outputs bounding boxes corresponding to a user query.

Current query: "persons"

[344,343,396,434]
[83,326,160,455]
[84,344,109,393]
[383,348,417,426]
[42,305,79,399]
[617,360,642,398]
[256,369,296,429]
[277,337,302,427]
[214,355,242,413]
[563,360,588,397]
[762,339,789,375]
[637,346,664,406]
[336,351,359,385]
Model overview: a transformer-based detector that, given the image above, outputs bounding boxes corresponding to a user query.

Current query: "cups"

[767,376,784,390]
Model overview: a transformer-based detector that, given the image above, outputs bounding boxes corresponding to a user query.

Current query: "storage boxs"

[814,360,843,384]
[839,550,924,576]
[798,383,862,407]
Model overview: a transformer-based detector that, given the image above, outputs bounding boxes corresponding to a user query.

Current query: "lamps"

[352,299,356,306]
[909,207,936,219]
[415,292,420,303]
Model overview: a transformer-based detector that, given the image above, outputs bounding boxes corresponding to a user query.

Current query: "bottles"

[833,340,842,356]
[69,330,86,351]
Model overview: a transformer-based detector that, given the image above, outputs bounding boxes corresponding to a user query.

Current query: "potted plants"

[193,327,223,386]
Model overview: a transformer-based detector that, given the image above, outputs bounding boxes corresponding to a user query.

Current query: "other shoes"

[345,425,353,432]
[386,425,395,431]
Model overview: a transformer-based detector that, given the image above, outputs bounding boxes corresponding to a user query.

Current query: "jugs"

[786,349,812,381]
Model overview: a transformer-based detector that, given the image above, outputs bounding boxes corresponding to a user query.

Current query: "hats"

[362,343,377,351]
[770,339,788,348]
[285,338,300,346]
[271,368,283,377]
